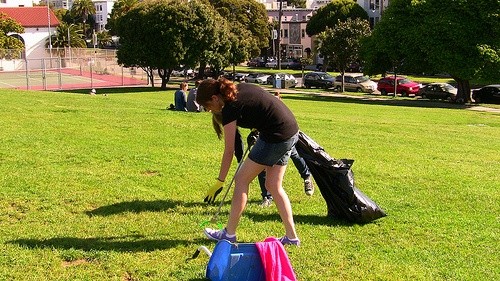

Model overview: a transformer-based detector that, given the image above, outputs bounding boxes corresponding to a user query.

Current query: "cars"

[316,63,367,72]
[170,65,271,85]
[264,59,278,68]
[418,83,458,102]
[280,59,301,69]
[246,58,265,67]
[472,85,500,105]
[267,74,299,87]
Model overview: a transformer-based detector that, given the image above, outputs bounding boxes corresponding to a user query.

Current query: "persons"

[174,82,188,111]
[203,102,314,208]
[185,79,205,112]
[195,79,300,247]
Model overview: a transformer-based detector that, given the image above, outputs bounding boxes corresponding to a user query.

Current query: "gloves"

[204,178,225,205]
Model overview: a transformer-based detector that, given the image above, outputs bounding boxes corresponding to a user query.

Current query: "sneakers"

[276,235,299,246]
[304,176,314,196]
[259,196,272,208]
[203,227,236,244]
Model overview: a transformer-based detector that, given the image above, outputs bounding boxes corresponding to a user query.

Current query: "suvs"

[302,72,336,90]
[377,76,420,97]
[333,74,378,94]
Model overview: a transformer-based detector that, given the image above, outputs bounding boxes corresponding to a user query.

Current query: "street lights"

[68,24,75,67]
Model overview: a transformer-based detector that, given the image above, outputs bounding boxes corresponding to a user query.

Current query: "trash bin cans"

[130,67,136,75]
[273,73,295,89]
